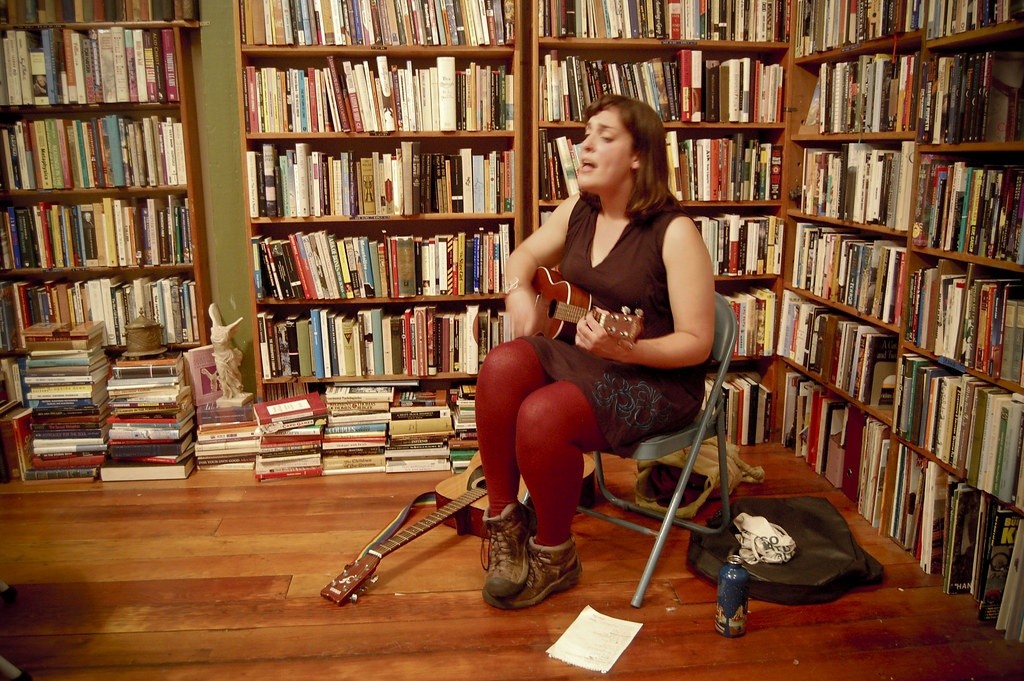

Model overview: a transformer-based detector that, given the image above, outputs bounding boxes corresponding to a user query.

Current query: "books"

[1,0,520,482]
[776,2,1024,681]
[537,0,787,445]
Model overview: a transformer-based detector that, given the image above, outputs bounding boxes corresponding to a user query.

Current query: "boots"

[481,503,535,598]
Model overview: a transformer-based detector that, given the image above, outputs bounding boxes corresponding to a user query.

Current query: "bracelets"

[501,277,533,301]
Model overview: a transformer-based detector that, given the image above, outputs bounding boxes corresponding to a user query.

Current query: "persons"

[480,95,716,608]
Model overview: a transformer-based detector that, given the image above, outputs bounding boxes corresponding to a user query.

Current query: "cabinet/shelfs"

[769,1,906,537]
[1,1,215,356]
[902,1,1023,638]
[232,1,529,408]
[530,2,794,445]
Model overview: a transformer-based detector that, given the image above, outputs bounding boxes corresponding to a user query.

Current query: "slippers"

[482,538,583,610]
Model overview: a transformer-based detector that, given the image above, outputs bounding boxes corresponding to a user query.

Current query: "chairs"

[520,292,739,609]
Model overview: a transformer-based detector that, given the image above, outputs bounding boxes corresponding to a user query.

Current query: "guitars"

[528,266,645,344]
[319,450,596,610]
